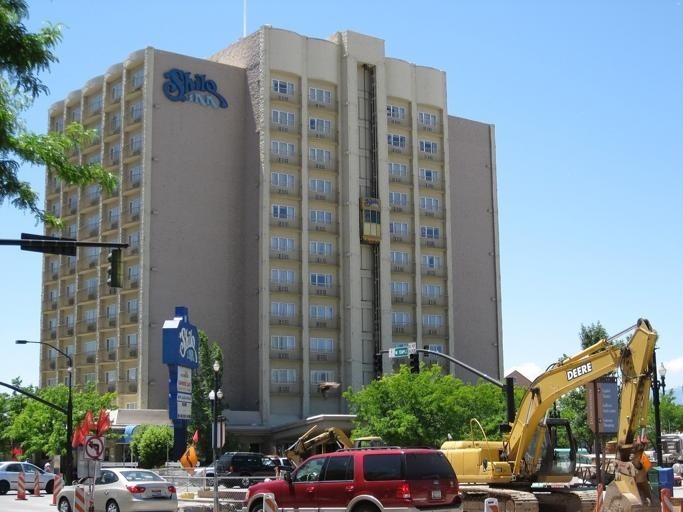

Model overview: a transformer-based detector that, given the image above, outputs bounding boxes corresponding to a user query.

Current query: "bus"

[660,433,682,467]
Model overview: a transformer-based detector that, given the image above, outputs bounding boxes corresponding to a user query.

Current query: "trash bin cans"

[648,466,673,497]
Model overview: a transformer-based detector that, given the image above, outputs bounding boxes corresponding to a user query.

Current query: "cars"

[56,468,178,512]
[0,461,55,495]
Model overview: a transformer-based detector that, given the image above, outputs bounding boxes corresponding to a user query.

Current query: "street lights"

[208,360,223,512]
[648,347,667,470]
[15,340,73,485]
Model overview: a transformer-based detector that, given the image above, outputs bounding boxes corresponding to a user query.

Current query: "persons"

[44,463,51,473]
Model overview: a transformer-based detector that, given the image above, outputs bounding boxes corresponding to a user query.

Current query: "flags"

[72,407,111,449]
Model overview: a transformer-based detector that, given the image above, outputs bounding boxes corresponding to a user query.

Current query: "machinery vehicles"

[283,425,386,470]
[438,319,657,512]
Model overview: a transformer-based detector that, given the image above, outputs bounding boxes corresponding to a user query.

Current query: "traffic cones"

[31,470,44,497]
[72,483,84,512]
[592,482,603,512]
[16,470,28,501]
[260,491,277,511]
[50,474,64,506]
[658,487,673,512]
[482,496,498,512]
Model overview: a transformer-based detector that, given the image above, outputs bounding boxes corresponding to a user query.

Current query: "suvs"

[188,460,220,488]
[217,451,293,488]
[240,446,463,512]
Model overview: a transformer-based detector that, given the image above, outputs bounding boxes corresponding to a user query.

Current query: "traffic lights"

[106,248,121,288]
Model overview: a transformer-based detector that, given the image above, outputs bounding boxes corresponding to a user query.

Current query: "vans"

[552,447,591,472]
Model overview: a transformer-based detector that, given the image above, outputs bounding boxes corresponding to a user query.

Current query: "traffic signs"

[387,341,416,359]
